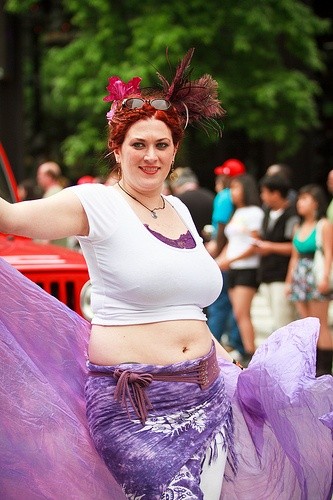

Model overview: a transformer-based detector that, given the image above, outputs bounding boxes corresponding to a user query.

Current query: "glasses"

[122,97,171,111]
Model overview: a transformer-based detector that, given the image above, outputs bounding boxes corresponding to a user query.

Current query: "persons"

[0,47,333,500]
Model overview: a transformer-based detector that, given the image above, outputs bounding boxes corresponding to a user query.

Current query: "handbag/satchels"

[314,217,332,295]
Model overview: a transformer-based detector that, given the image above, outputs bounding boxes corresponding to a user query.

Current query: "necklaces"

[118,181,165,219]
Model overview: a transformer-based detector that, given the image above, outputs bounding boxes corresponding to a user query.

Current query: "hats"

[215,158,245,177]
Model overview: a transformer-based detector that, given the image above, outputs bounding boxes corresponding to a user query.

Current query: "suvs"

[0,142,91,322]
